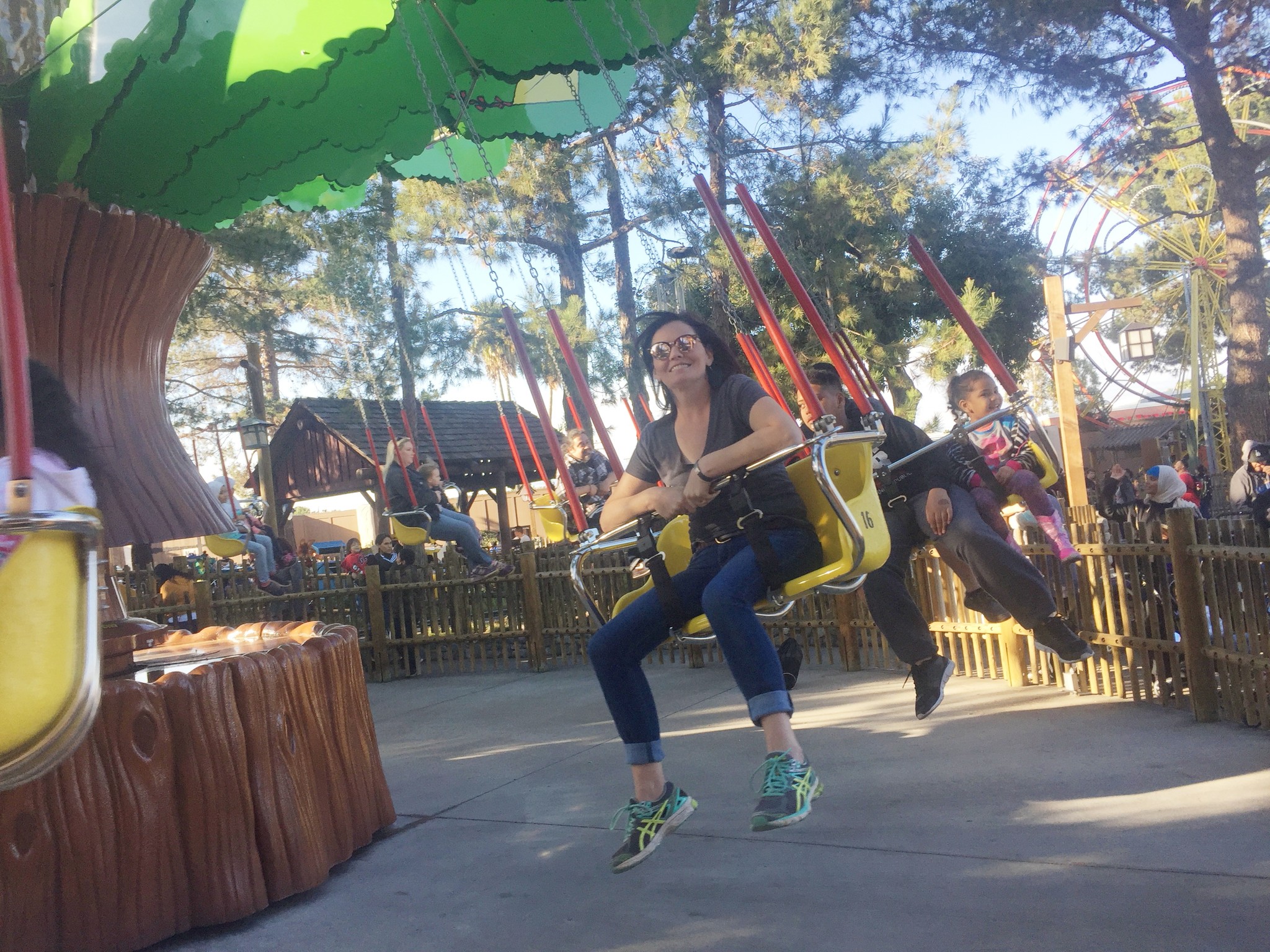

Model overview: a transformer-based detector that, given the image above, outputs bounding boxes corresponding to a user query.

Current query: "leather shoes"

[609,781,698,872]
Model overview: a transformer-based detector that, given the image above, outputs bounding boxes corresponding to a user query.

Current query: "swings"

[424,180,621,545]
[391,0,891,650]
[1,124,105,792]
[527,0,1064,549]
[190,415,271,560]
[312,208,463,548]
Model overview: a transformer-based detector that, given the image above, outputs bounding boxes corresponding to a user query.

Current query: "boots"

[1005,534,1046,579]
[1035,510,1084,564]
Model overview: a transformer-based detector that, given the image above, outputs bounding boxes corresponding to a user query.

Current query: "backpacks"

[1183,472,1205,498]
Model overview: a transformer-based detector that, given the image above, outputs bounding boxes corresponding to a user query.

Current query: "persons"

[589,312,823,870]
[943,370,1084,576]
[554,429,650,580]
[798,362,1095,719]
[109,437,1270,699]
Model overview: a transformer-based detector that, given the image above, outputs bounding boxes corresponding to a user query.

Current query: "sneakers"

[749,742,824,832]
[490,559,516,577]
[469,566,499,583]
[1032,615,1093,664]
[901,653,956,719]
[963,586,1009,623]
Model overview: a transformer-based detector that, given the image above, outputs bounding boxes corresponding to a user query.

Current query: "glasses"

[647,334,701,361]
[396,436,404,442]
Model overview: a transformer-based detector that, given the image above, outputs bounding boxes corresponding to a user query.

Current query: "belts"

[696,531,737,551]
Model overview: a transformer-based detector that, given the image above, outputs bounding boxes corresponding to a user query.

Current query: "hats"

[1248,445,1269,463]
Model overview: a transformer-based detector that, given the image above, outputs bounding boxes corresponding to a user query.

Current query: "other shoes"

[270,574,289,586]
[409,659,417,676]
[400,658,406,669]
[365,660,375,672]
[258,580,285,597]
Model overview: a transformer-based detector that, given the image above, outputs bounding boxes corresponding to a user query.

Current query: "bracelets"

[694,460,715,482]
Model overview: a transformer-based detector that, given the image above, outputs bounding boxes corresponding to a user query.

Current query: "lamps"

[1027,347,1051,362]
[1117,315,1157,364]
